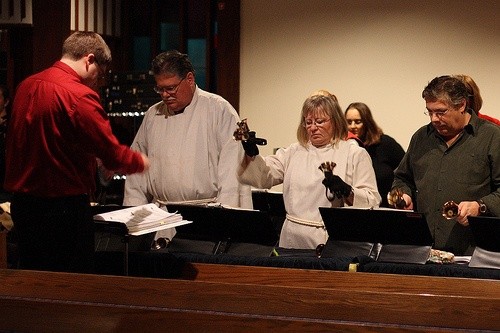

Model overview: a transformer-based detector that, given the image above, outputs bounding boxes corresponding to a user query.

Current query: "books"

[94,203,193,235]
[251,189,283,194]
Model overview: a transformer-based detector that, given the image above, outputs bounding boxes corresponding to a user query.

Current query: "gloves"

[241,130,259,156]
[322,171,351,199]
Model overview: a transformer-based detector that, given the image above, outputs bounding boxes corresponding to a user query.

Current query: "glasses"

[153,75,187,94]
[95,58,106,81]
[424,105,454,118]
[301,118,331,127]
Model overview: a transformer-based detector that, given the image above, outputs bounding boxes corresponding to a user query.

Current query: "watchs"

[478,200,488,217]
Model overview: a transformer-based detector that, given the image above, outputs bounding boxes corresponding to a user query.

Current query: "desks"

[0,215,500,333]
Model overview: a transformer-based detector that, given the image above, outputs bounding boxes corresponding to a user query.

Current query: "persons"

[121,51,253,211]
[388,74,500,256]
[233,90,382,250]
[0,31,150,274]
[342,103,406,209]
